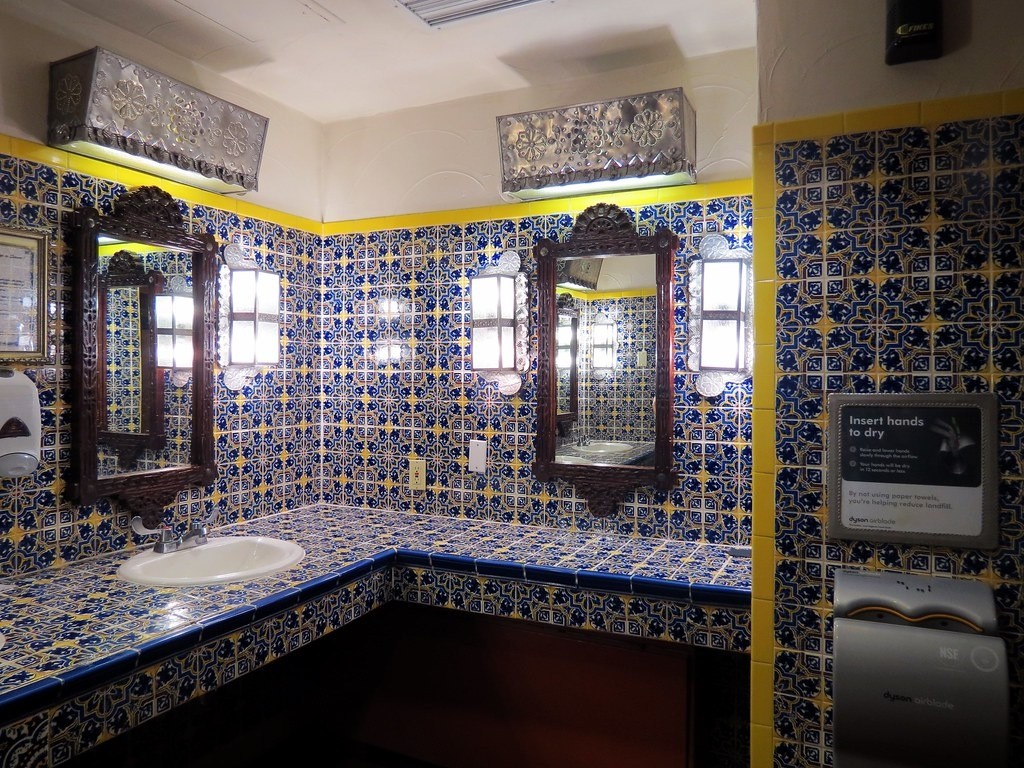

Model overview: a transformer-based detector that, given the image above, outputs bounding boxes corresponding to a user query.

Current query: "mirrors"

[67,186,218,531]
[555,293,581,434]
[536,205,679,519]
[98,249,166,469]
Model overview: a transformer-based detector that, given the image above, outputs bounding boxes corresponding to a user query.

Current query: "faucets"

[583,434,593,446]
[174,527,211,551]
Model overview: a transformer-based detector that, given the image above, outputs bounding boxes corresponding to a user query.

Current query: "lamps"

[496,86,697,201]
[470,251,531,396]
[46,47,270,195]
[154,276,196,386]
[682,236,755,398]
[557,258,605,293]
[217,244,279,390]
[591,314,617,380]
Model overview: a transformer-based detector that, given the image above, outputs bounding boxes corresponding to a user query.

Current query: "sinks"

[572,442,633,453]
[116,535,306,587]
